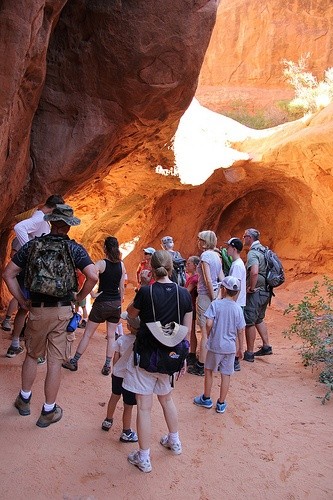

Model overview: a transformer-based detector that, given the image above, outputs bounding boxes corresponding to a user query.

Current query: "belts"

[32,302,71,307]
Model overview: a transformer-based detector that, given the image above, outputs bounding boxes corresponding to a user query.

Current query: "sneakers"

[254,346,272,355]
[216,400,227,412]
[244,351,254,362]
[37,355,46,364]
[186,353,205,375]
[120,431,138,442]
[127,449,152,473]
[15,393,32,415]
[194,394,213,408]
[78,320,87,328]
[7,345,24,358]
[102,420,112,431]
[102,365,111,375]
[36,407,63,427]
[62,361,77,371]
[160,433,182,454]
[234,362,240,371]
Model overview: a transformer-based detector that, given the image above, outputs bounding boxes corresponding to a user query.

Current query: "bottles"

[67,312,82,332]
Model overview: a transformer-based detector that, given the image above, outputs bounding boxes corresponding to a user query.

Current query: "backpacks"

[264,249,285,287]
[140,270,152,286]
[222,248,232,277]
[172,258,186,286]
[139,337,189,376]
[24,236,77,297]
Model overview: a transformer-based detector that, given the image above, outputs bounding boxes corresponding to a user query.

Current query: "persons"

[137,247,156,288]
[1,237,23,331]
[160,236,186,287]
[6,193,65,364]
[73,267,88,328]
[226,237,246,371]
[1,204,98,428]
[127,250,192,472]
[184,256,200,370]
[213,237,222,264]
[193,276,246,413]
[242,228,273,362]
[105,252,124,340]
[62,236,124,376]
[188,231,226,375]
[102,314,139,442]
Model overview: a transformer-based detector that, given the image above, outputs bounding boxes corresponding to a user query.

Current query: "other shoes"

[1,320,11,331]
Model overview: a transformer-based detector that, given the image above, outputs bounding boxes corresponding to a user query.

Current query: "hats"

[225,238,243,248]
[219,276,241,290]
[44,204,81,226]
[146,321,189,347]
[144,247,156,255]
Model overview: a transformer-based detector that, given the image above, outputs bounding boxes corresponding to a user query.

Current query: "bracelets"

[248,287,255,292]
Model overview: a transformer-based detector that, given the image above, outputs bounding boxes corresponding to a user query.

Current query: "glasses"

[243,235,251,238]
